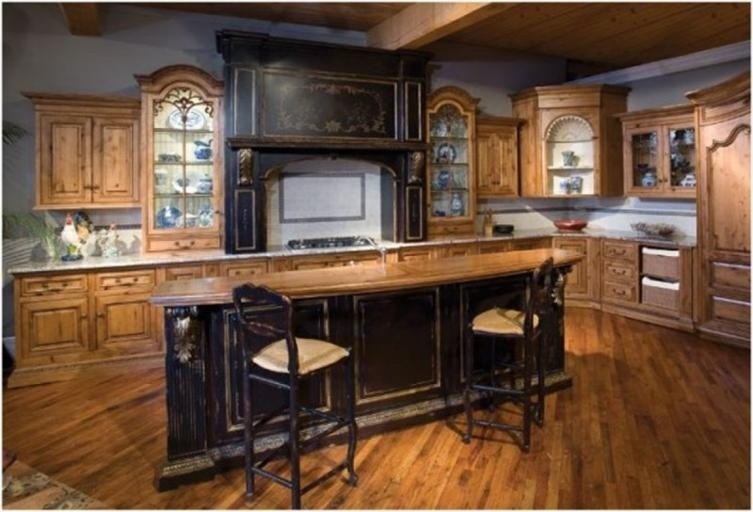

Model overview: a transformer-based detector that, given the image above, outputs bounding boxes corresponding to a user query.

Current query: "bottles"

[640,171,655,188]
[196,173,212,195]
[680,173,696,188]
[450,193,461,216]
[437,170,449,190]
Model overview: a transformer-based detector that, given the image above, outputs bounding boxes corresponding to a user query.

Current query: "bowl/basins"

[493,224,513,234]
[157,152,180,162]
[553,219,587,232]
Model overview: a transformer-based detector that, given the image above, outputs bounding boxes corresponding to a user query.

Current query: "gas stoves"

[286,235,376,250]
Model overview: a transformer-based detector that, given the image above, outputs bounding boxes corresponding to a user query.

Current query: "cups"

[561,150,574,167]
[559,178,569,195]
[570,175,582,194]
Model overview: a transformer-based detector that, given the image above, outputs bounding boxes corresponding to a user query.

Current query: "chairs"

[230,282,361,510]
[458,253,555,453]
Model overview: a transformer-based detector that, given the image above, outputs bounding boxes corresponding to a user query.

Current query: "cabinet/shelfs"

[8,271,159,373]
[425,86,697,228]
[20,83,142,212]
[137,61,227,255]
[684,67,747,349]
[550,235,695,337]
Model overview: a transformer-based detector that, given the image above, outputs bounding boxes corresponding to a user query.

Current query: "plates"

[436,142,457,163]
[172,177,197,195]
[156,206,182,228]
[166,106,207,131]
